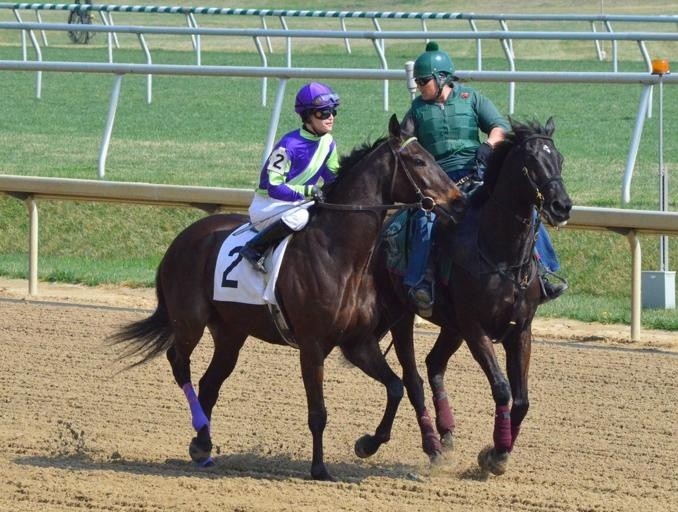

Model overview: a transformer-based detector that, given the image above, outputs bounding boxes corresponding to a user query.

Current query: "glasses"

[295,94,341,107]
[309,108,336,120]
[414,74,444,86]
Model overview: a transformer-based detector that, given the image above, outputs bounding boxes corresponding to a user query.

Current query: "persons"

[399,41,569,319]
[237,82,340,274]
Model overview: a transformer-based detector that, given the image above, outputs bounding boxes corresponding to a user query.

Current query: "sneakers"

[541,282,568,303]
[414,287,434,318]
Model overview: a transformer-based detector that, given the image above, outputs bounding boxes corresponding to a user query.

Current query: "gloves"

[476,142,492,163]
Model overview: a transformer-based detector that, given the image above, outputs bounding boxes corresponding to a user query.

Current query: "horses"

[124,113,463,479]
[378,113,574,473]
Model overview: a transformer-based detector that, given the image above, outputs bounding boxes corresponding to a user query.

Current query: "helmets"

[413,42,459,82]
[295,83,340,113]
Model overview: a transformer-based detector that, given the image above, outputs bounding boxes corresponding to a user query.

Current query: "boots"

[239,218,296,274]
